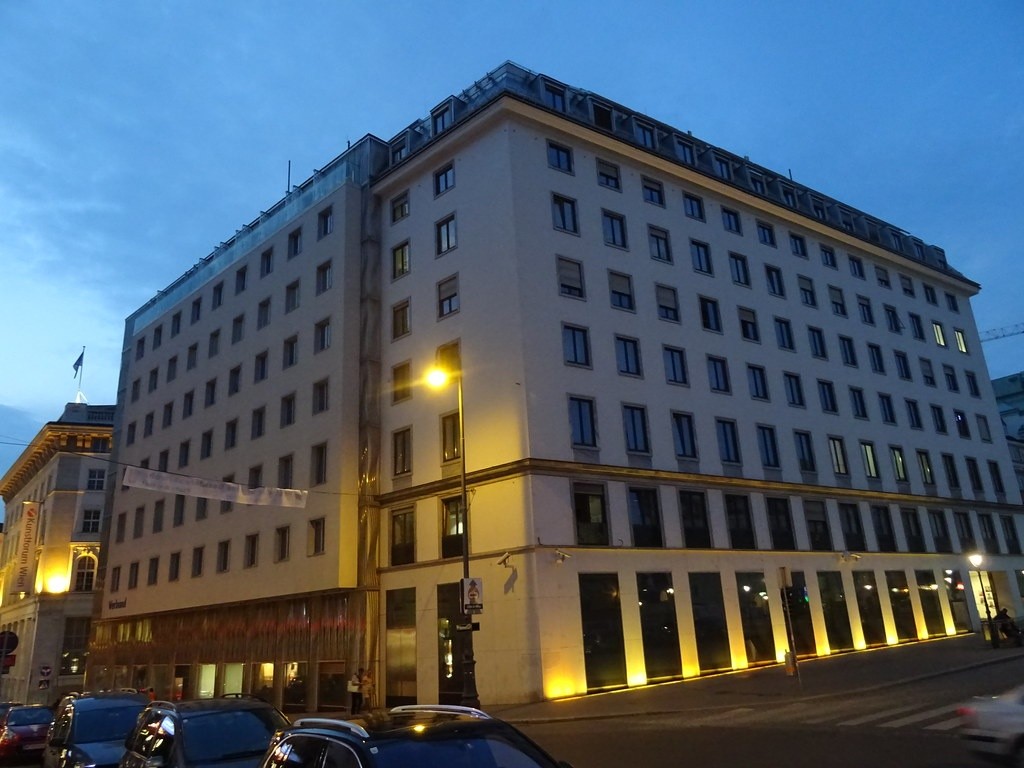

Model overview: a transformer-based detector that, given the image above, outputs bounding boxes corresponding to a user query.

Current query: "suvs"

[118,693,302,768]
[40,687,155,768]
[258,703,574,768]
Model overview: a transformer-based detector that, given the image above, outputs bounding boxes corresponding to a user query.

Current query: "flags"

[73,353,83,378]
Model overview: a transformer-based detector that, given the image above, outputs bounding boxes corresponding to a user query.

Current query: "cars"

[0,704,57,768]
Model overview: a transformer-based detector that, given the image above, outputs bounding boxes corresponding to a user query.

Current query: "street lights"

[968,554,999,648]
[426,366,481,710]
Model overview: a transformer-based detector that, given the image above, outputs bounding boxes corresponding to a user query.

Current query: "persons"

[352,667,374,714]
[993,608,1011,639]
[148,688,156,701]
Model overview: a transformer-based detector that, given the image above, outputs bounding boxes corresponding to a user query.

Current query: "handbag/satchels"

[347,680,359,692]
